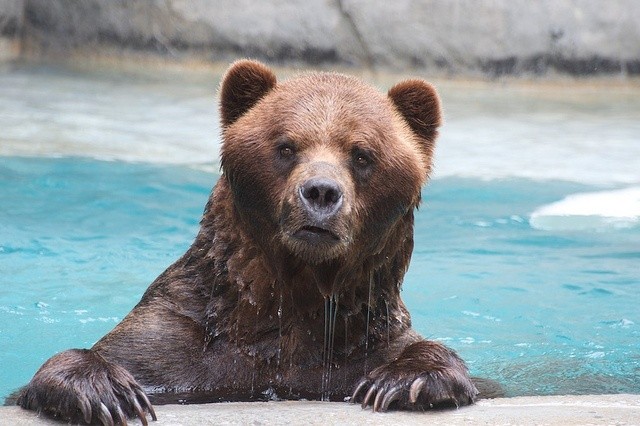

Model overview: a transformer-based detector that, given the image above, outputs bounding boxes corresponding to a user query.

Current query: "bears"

[1,60,499,426]
